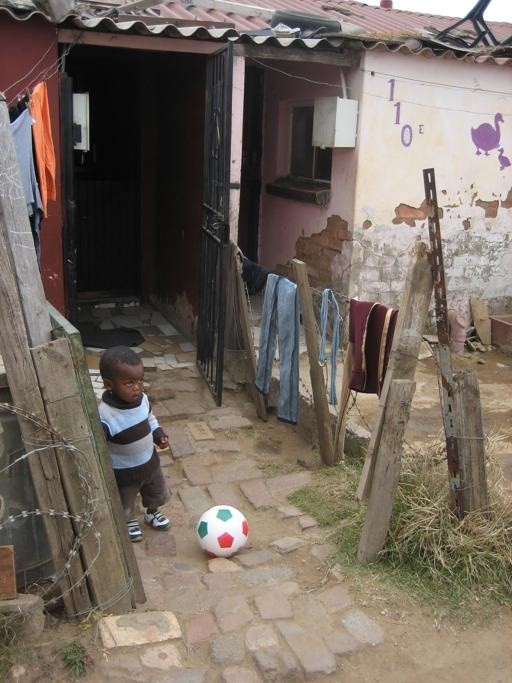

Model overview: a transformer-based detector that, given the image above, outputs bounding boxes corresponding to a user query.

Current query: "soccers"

[196,504,248,557]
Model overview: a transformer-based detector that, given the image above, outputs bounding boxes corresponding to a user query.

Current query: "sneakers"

[125,518,143,542]
[145,508,171,531]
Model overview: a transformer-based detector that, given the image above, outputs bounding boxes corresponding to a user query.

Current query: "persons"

[95,346,171,545]
